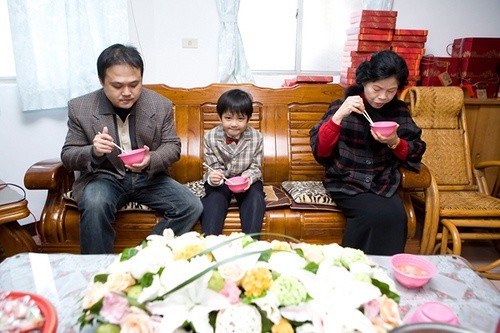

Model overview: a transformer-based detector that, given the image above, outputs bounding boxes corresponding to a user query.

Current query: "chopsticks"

[202,163,234,184]
[362,110,375,126]
[97,131,129,154]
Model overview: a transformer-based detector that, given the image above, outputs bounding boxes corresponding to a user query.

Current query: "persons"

[309,50,426,256]
[60,44,204,255]
[199,89,264,241]
[131,255,132,255]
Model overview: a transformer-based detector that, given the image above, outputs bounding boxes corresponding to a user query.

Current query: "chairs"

[399,86,500,280]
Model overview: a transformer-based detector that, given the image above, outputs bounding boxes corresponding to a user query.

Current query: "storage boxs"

[280,10,500,99]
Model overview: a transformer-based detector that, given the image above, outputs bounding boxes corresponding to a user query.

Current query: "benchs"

[22,83,431,254]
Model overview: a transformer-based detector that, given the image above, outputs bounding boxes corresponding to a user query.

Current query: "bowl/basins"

[390,254,437,288]
[118,148,146,167]
[224,176,249,193]
[370,121,397,136]
[406,301,462,326]
[0,291,58,333]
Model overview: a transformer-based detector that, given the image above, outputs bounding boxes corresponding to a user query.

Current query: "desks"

[0,179,37,263]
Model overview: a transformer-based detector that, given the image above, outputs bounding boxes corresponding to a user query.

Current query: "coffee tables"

[0,251,500,333]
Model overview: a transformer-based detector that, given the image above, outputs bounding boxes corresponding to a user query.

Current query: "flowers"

[74,228,404,333]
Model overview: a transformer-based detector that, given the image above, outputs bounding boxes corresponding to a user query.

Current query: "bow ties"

[225,136,238,145]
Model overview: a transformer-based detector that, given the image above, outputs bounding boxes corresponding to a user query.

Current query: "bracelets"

[387,136,400,149]
